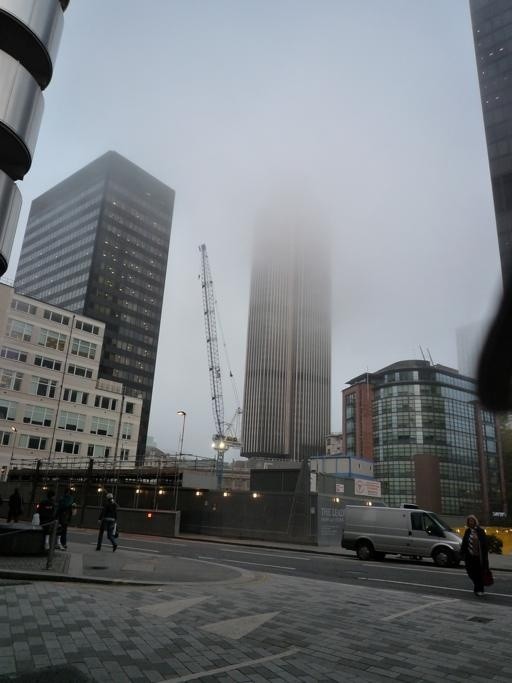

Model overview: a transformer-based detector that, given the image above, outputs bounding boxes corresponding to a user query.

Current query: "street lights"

[177,410,187,453]
[9,424,17,460]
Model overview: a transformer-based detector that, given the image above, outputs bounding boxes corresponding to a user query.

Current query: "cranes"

[199,243,241,488]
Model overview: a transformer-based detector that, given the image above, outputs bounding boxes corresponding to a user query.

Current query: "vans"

[340,504,463,568]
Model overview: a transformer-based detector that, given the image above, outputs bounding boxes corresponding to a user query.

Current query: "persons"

[57,488,73,548]
[459,514,493,596]
[96,493,119,552]
[39,491,74,550]
[6,486,21,523]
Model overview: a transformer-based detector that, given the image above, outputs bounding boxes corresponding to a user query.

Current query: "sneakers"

[96,544,101,550]
[45,542,67,551]
[113,543,117,551]
[476,592,483,596]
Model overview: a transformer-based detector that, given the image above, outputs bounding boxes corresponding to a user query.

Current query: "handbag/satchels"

[481,565,493,585]
[112,526,119,538]
